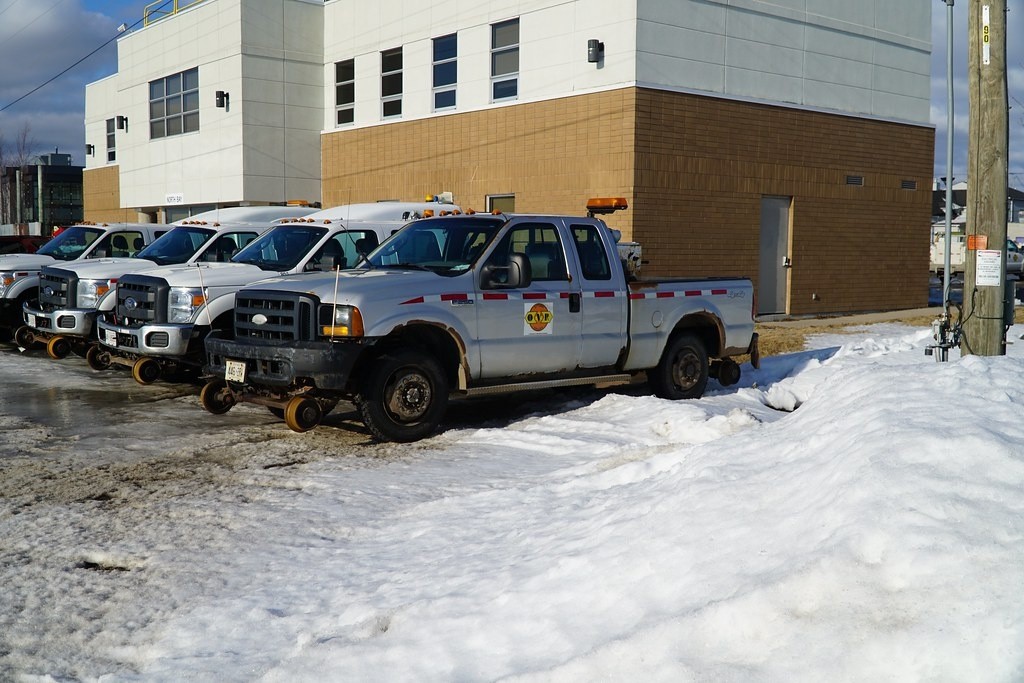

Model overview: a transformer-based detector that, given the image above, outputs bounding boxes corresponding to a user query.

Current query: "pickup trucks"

[86,190,645,384]
[17,199,324,359]
[0,220,177,342]
[196,197,760,444]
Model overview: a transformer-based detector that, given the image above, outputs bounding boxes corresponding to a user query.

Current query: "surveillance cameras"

[117,23,127,32]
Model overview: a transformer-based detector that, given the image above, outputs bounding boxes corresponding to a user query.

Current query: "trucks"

[929,234,1023,281]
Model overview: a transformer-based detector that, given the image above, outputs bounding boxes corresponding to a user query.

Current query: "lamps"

[588,40,603,64]
[85,143,91,154]
[117,116,128,129]
[216,91,227,107]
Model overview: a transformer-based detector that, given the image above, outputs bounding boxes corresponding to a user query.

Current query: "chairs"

[467,246,482,261]
[131,238,144,258]
[579,243,603,276]
[319,238,347,269]
[351,238,377,269]
[111,235,129,257]
[206,237,239,261]
[525,244,564,280]
